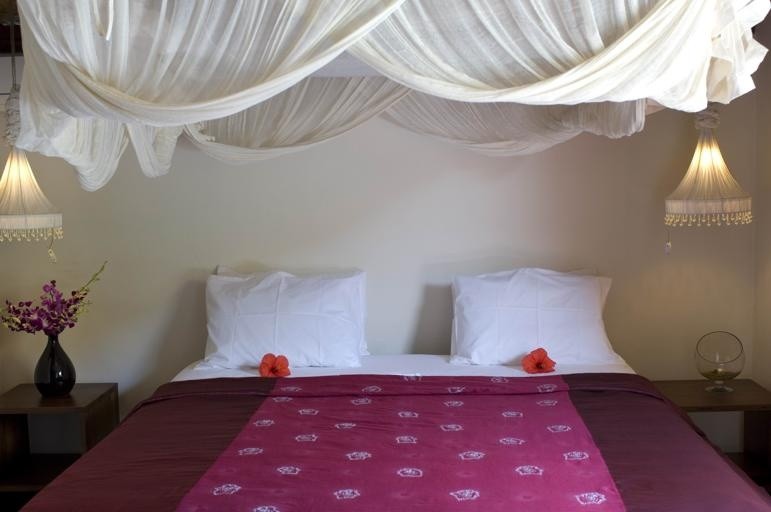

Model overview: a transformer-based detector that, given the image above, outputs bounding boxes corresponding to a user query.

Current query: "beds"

[19,274,771,512]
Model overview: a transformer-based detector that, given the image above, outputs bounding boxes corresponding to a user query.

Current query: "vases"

[35,333,76,398]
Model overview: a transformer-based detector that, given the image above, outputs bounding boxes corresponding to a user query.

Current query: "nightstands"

[1,383,120,510]
[649,380,771,495]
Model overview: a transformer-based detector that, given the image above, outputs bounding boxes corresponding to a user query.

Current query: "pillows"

[447,266,626,366]
[191,266,370,370]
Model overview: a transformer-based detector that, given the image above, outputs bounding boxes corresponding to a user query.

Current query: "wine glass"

[694,331,746,394]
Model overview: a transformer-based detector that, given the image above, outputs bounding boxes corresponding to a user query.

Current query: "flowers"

[2,262,110,335]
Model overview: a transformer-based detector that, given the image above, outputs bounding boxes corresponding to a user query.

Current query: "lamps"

[666,104,753,227]
[0,0,64,242]
